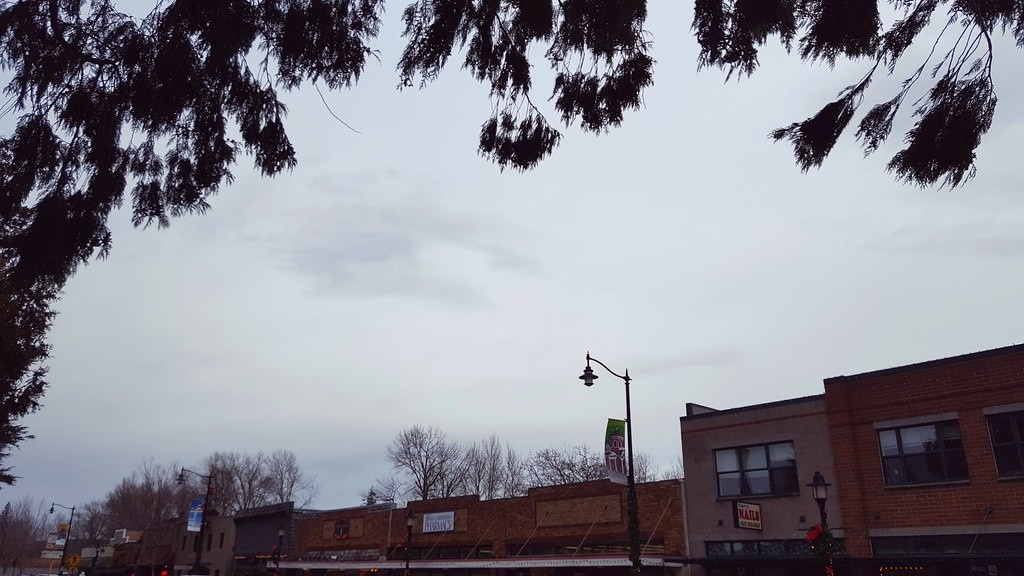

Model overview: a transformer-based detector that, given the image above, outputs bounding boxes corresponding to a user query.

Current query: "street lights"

[805,470,835,576]
[404,509,418,576]
[274,524,286,576]
[176,467,213,576]
[95,542,102,559]
[577,349,642,576]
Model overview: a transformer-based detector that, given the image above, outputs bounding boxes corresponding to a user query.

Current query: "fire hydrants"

[48,502,77,576]
